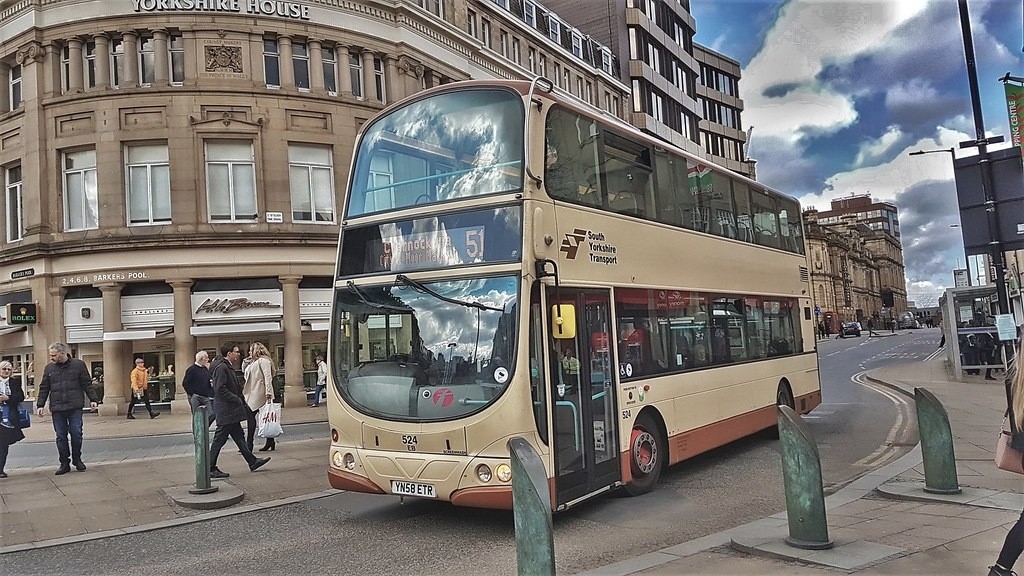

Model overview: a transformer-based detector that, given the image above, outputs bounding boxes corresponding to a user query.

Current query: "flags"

[687,159,713,196]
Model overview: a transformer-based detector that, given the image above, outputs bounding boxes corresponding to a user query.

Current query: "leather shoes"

[249,457,271,471]
[209,467,229,478]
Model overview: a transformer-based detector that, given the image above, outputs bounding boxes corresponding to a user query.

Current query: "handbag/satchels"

[18,409,30,428]
[258,397,284,438]
[318,387,327,403]
[995,429,1024,474]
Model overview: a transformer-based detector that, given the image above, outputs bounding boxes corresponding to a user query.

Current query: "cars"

[843,322,860,337]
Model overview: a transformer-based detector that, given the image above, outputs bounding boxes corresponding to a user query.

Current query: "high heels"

[259,441,275,452]
[237,442,253,452]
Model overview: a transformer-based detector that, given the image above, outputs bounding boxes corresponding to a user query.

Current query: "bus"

[326,77,822,519]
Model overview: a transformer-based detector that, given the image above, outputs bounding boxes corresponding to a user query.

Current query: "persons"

[987,325,1024,576]
[775,335,790,349]
[866,317,876,337]
[238,342,276,453]
[0,361,24,478]
[824,316,829,338]
[938,319,945,348]
[126,358,160,419]
[91,367,104,403]
[891,318,896,333]
[309,355,326,407]
[563,349,581,394]
[685,344,718,368]
[37,342,99,475]
[957,308,1005,380]
[926,317,933,328]
[819,321,825,339]
[208,341,271,478]
[147,366,158,400]
[166,364,175,400]
[183,352,215,429]
[835,321,846,339]
[622,312,660,374]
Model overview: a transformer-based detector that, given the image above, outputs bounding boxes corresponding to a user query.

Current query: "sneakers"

[1,420,15,429]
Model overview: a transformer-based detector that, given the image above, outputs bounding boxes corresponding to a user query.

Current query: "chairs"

[579,181,790,253]
[413,194,432,205]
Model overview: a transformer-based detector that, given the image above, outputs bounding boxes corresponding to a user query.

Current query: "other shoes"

[987,565,1018,576]
[0,471,7,478]
[149,411,160,419]
[127,413,135,419]
[73,461,86,470]
[55,465,70,475]
[311,403,319,407]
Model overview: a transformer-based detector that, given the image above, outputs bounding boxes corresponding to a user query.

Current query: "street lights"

[908,146,973,287]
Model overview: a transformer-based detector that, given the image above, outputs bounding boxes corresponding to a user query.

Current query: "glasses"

[233,350,240,354]
[137,362,144,364]
[1,368,11,371]
